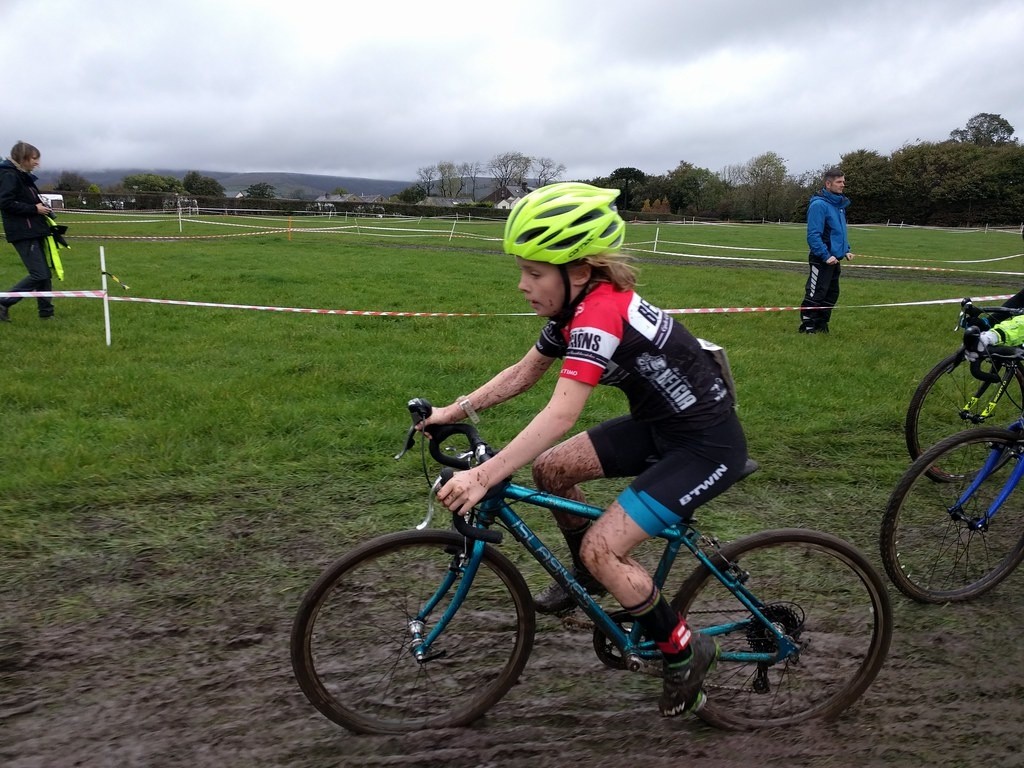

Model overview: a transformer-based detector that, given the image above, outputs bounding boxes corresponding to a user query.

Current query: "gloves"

[964,330,998,362]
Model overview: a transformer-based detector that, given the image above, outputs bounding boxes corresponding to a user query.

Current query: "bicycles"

[289,398,895,739]
[879,324,1024,604]
[905,297,1024,485]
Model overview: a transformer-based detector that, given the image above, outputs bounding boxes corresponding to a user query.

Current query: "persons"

[411,181,749,723]
[0,141,57,324]
[960,287,1023,334]
[963,310,1024,362]
[798,169,854,334]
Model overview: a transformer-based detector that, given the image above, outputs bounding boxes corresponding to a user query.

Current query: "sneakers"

[0,301,10,324]
[531,568,604,613]
[656,635,719,723]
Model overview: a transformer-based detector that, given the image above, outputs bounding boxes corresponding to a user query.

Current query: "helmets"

[501,181,625,265]
